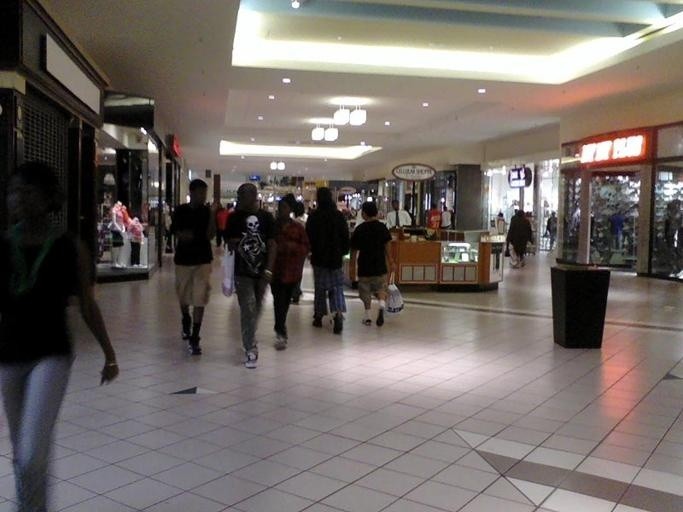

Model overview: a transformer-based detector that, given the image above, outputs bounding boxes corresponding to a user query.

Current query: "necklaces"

[7,219,62,296]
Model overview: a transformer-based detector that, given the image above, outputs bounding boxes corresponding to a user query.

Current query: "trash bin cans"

[551,265,611,348]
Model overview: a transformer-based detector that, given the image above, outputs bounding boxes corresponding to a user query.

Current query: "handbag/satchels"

[387,284,403,312]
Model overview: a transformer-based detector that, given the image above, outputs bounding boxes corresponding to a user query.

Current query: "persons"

[0,159,120,512]
[108,197,145,268]
[497,208,556,270]
[608,208,627,251]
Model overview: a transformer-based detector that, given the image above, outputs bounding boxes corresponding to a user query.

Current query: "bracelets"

[103,362,119,368]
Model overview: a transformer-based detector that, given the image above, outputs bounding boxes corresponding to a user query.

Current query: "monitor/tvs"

[507,167,532,188]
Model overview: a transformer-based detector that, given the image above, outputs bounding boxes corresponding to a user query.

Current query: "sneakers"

[181,318,201,354]
[363,319,371,325]
[245,353,258,367]
[377,309,383,326]
